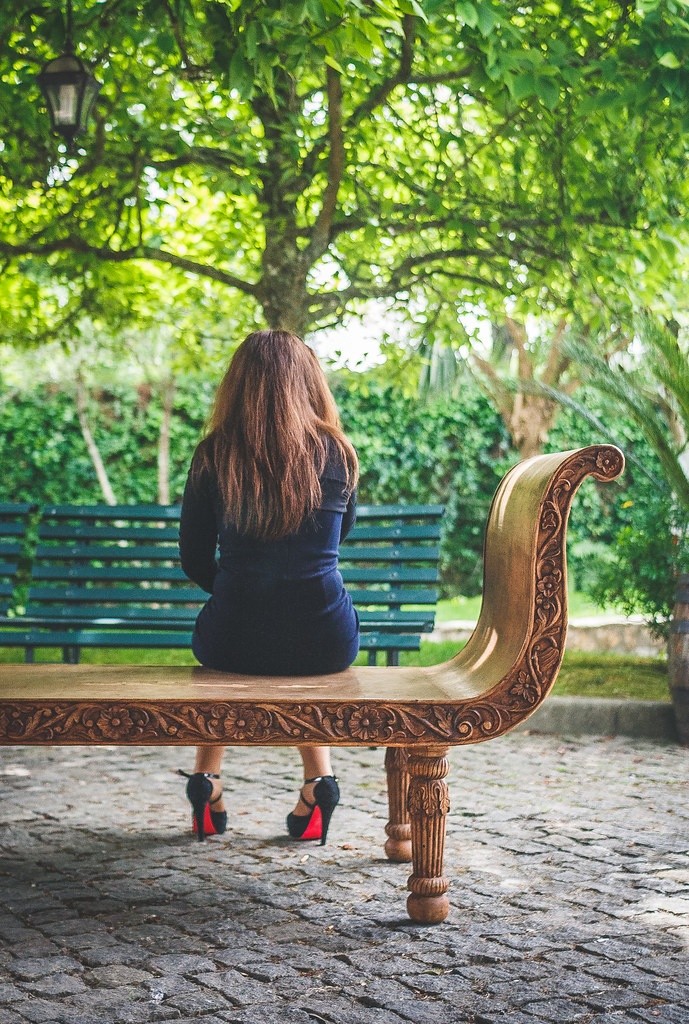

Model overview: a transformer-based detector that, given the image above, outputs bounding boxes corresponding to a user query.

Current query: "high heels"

[286,775,340,845]
[177,769,227,842]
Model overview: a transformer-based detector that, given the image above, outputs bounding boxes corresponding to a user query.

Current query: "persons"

[179,331,361,844]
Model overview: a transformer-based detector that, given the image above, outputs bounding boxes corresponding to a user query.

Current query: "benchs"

[0,503,443,750]
[0,504,37,613]
[0,441,625,927]
